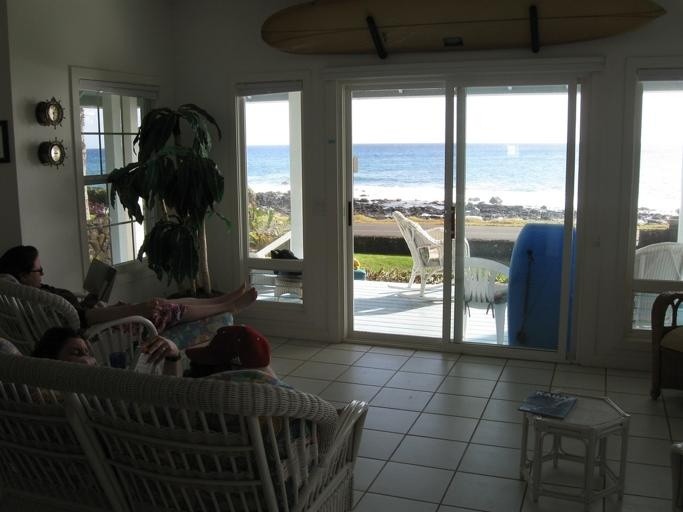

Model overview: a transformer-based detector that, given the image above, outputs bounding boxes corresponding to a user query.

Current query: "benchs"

[0,351,370,510]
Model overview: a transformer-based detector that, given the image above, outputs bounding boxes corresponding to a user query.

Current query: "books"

[83,257,116,303]
[518,389,577,420]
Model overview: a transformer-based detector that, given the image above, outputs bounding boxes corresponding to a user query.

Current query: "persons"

[28,325,180,422]
[0,245,258,332]
[183,325,277,379]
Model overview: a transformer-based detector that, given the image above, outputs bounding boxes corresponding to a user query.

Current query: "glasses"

[31,267,43,275]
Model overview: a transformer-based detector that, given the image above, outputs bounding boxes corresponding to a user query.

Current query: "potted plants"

[103,102,231,298]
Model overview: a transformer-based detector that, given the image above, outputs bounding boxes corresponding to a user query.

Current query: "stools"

[157,310,234,376]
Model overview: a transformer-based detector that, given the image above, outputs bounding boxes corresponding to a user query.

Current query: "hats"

[184,325,272,369]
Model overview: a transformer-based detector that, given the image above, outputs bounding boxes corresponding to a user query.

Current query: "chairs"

[505,222,576,356]
[647,290,682,401]
[0,274,158,370]
[451,252,512,345]
[391,208,469,298]
[633,242,682,328]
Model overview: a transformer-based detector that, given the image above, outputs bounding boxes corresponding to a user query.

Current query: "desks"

[518,387,629,511]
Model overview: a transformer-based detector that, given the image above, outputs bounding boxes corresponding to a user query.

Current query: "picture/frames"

[0,120,10,164]
